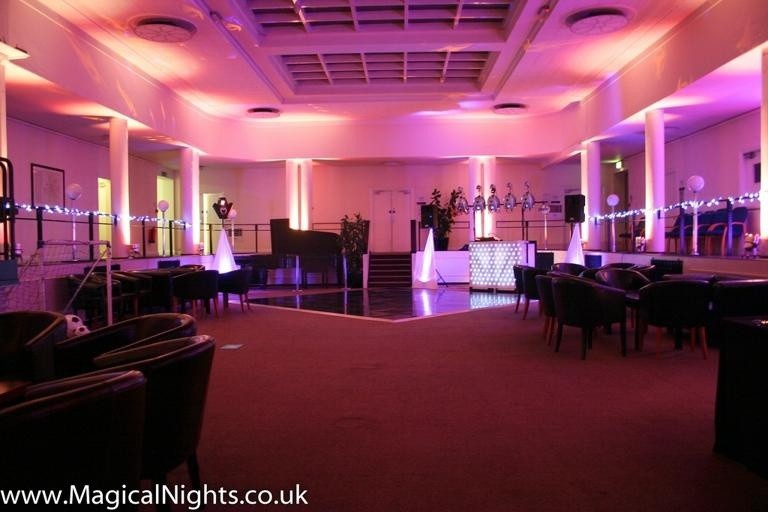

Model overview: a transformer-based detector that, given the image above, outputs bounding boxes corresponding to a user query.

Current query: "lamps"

[540,204,551,250]
[157,200,169,257]
[686,175,705,256]
[606,194,620,252]
[228,208,237,249]
[65,183,83,262]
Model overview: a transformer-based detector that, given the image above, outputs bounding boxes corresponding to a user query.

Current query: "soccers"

[65,315,91,339]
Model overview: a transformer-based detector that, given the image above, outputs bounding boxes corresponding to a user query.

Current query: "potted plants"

[334,210,367,289]
[425,187,463,251]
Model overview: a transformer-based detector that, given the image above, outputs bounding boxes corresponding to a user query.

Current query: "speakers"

[564,194,585,222]
[421,205,438,227]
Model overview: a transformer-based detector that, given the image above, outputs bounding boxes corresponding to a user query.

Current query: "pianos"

[234,219,341,289]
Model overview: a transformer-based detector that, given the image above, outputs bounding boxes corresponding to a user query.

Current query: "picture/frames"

[29,162,66,210]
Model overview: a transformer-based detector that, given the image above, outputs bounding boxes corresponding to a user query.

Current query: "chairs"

[68,263,253,317]
[512,262,768,363]
[618,206,749,258]
